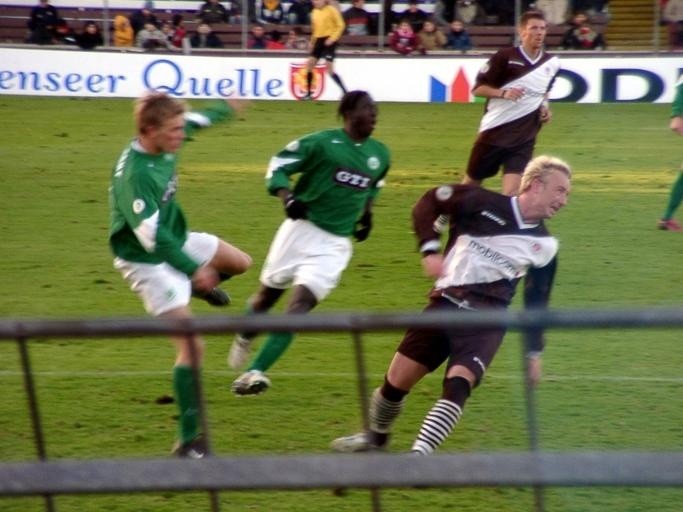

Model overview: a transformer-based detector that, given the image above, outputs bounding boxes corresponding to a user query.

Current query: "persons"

[106,90,251,459]
[228,91,391,395]
[297,0,349,103]
[181,95,250,140]
[328,154,570,455]
[658,63,683,232]
[384,0,487,54]
[536,0,612,50]
[342,0,376,36]
[112,1,229,51]
[248,1,308,52]
[432,12,562,236]
[23,0,102,50]
[660,1,683,51]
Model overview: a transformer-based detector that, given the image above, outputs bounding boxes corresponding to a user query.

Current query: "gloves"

[355,211,371,242]
[284,195,308,221]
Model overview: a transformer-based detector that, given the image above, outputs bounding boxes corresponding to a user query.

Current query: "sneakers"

[228,333,250,368]
[331,432,380,452]
[173,438,216,459]
[233,370,269,394]
[658,219,683,231]
[301,95,311,101]
[191,286,230,307]
